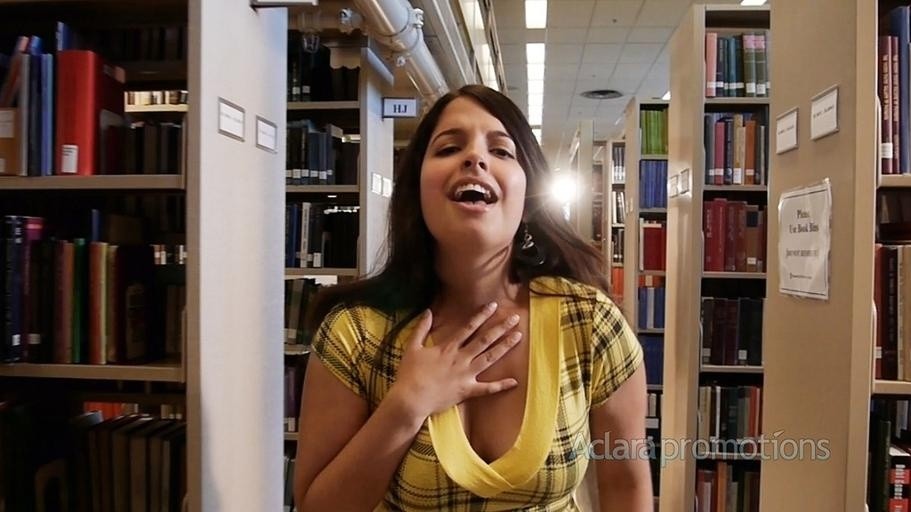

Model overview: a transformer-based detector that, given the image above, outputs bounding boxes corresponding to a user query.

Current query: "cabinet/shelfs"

[758,0,911,512]
[599,139,625,304]
[285,27,394,512]
[592,140,606,242]
[0,1,287,512]
[622,99,669,511]
[658,4,770,511]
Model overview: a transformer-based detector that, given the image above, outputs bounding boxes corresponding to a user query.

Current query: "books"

[592,25,911,512]
[0,16,360,511]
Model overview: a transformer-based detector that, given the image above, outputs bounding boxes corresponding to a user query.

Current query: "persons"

[294,84,655,511]
